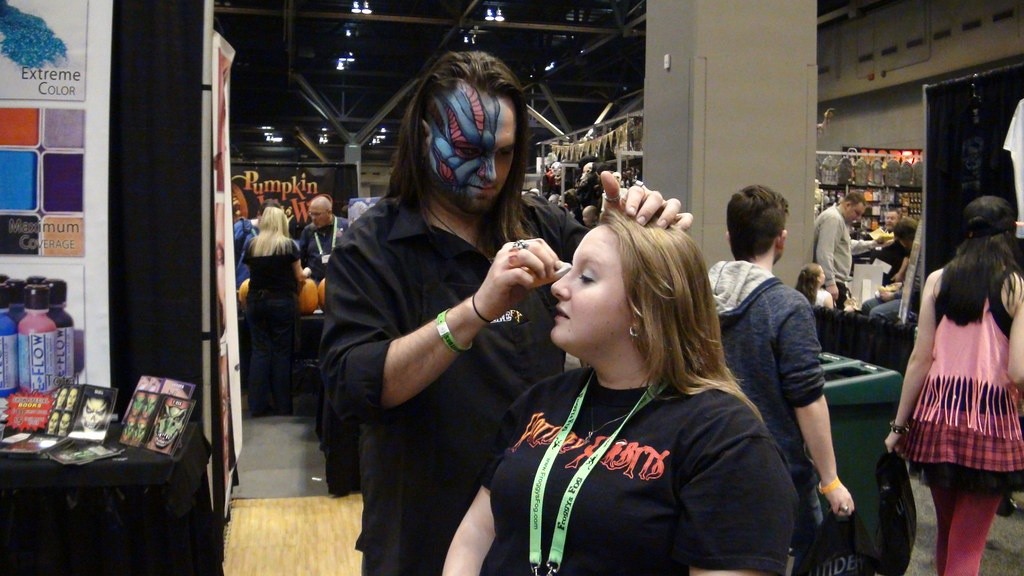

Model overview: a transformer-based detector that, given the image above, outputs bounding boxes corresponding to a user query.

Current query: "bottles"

[1,273,74,403]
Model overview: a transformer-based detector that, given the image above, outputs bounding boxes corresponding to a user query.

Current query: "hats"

[960,194,1017,239]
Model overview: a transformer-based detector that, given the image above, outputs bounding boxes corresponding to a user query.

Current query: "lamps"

[494,1,506,22]
[485,0,494,21]
[351,0,373,15]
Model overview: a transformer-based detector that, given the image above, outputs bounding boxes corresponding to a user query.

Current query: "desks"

[292,312,327,416]
[0,421,226,576]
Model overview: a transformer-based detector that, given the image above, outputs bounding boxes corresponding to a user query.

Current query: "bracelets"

[472,292,494,323]
[816,476,840,495]
[436,308,473,353]
[889,420,910,433]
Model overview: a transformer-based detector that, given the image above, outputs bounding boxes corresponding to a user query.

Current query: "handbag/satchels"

[874,447,918,576]
[790,507,883,575]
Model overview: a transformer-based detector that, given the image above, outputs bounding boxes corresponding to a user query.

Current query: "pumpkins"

[238,278,326,315]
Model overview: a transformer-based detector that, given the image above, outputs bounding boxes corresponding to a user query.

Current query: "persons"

[879,195,1024,576]
[812,191,897,312]
[698,182,857,574]
[232,198,283,306]
[867,203,904,262]
[795,263,835,311]
[314,49,691,575]
[441,208,802,574]
[239,206,307,418]
[298,195,356,284]
[860,215,920,319]
[521,185,604,227]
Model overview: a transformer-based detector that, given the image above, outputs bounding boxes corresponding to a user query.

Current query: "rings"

[842,506,849,513]
[603,193,622,203]
[511,238,530,250]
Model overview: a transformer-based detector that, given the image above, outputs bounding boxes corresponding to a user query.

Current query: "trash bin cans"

[812,352,902,570]
